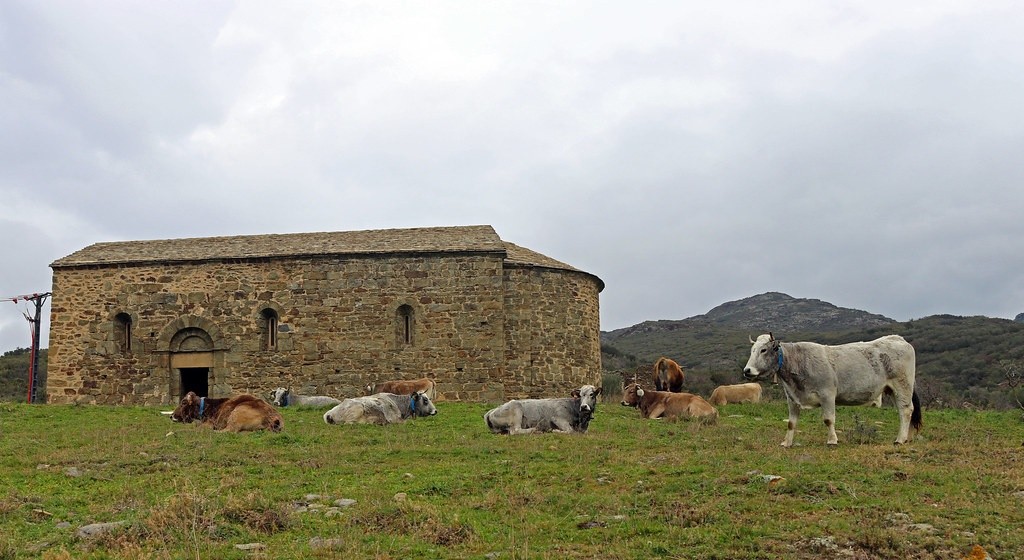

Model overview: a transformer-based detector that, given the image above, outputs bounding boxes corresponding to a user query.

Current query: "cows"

[171,391,284,432]
[483,385,603,435]
[743,332,923,446]
[270,378,437,426]
[621,358,763,423]
